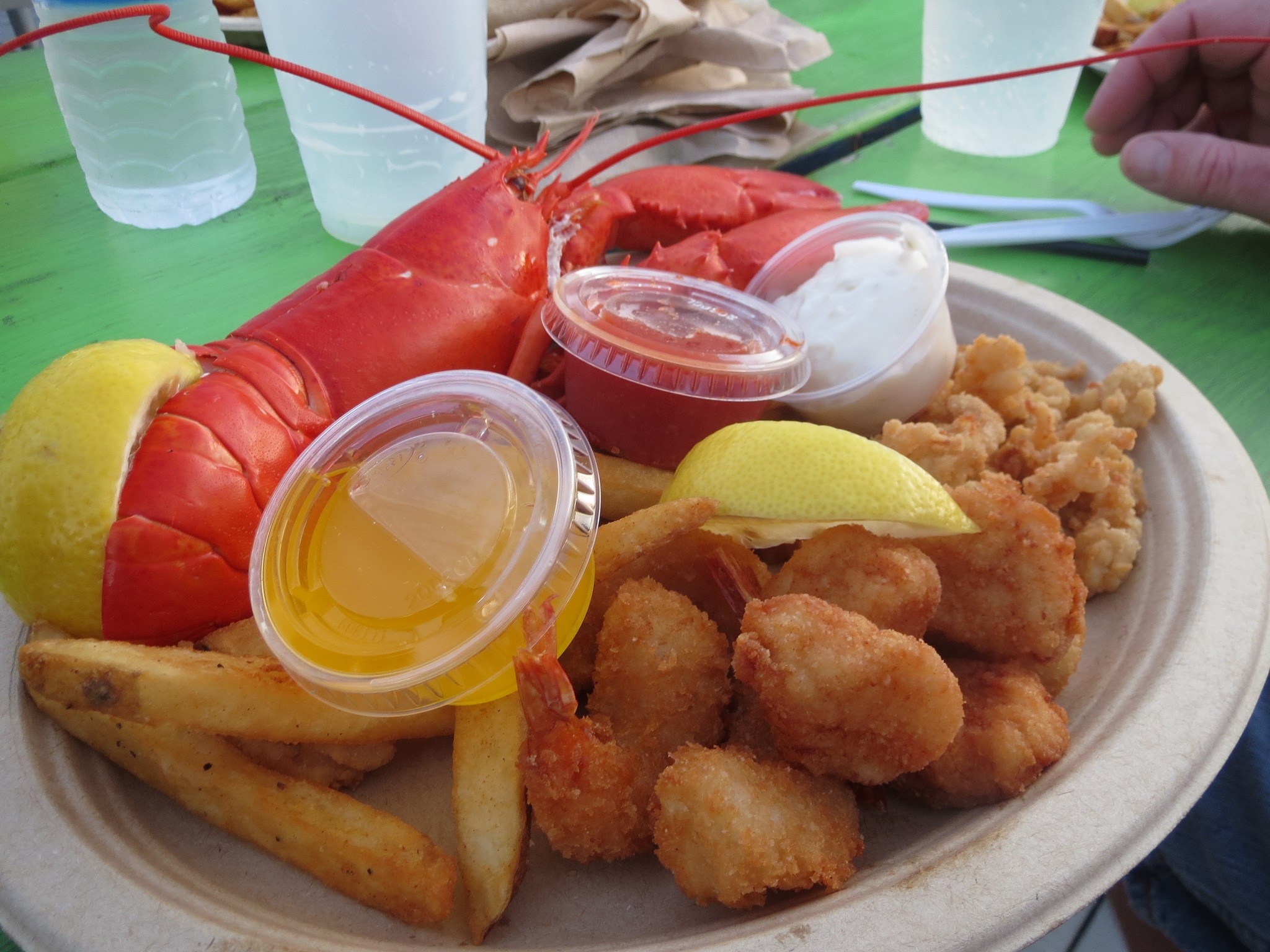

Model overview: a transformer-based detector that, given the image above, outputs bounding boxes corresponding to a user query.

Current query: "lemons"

[659,420,980,544]
[2,336,204,640]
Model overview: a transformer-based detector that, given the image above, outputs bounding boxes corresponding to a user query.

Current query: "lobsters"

[0,5,1270,645]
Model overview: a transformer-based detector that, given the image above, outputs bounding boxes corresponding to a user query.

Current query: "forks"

[850,181,1232,247]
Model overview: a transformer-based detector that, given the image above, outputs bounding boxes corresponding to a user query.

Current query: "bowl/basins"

[249,368,602,720]
[743,211,958,440]
[538,264,815,472]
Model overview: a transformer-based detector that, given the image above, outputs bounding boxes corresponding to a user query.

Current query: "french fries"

[19,445,687,940]
[1090,0,1183,53]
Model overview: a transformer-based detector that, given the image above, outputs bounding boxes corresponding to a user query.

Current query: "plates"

[1084,43,1124,78]
[1,234,1270,952]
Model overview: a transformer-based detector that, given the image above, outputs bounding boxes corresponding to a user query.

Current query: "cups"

[253,0,489,248]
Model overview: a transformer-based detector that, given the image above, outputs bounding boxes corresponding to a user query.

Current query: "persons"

[1085,0,1270,226]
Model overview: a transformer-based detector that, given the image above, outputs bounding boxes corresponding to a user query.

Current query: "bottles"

[919,0,1109,159]
[28,1,258,229]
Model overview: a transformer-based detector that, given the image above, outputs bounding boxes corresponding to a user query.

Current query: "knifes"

[936,208,1200,249]
